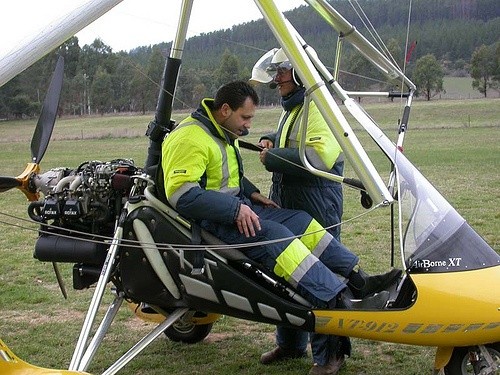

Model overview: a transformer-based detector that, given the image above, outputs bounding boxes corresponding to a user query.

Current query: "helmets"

[271,45,318,87]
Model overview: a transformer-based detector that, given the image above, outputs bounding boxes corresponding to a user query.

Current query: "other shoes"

[328,290,390,310]
[260,346,307,364]
[307,355,345,375]
[346,267,402,298]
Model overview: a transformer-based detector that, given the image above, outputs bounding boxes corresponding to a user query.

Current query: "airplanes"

[0,0,500,374]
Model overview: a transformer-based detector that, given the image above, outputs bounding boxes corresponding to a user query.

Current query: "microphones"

[270,79,293,89]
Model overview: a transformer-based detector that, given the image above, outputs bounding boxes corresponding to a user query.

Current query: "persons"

[250,47,351,374]
[161,78,401,308]
